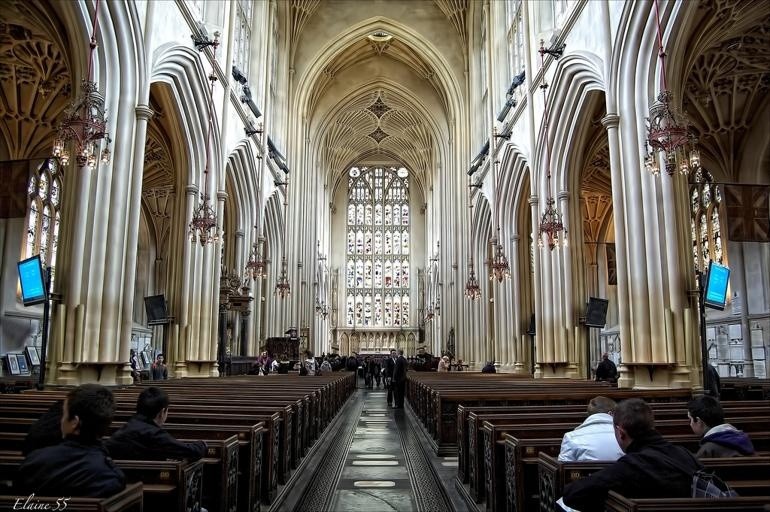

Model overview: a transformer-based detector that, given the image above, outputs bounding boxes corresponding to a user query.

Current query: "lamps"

[486,127,513,284]
[464,179,483,302]
[189,30,223,247]
[273,173,291,298]
[534,39,569,252]
[53,0,112,170]
[243,122,267,280]
[643,1,699,179]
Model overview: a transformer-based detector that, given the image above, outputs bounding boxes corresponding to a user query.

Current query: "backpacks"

[647,448,738,498]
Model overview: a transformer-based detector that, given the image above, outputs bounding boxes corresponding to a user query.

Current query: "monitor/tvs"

[17,254,48,307]
[583,297,609,328]
[703,259,730,311]
[144,294,169,326]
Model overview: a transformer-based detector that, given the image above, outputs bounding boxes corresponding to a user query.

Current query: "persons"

[558,351,770,512]
[255,349,497,408]
[110,387,209,464]
[150,354,168,380]
[12,384,126,499]
